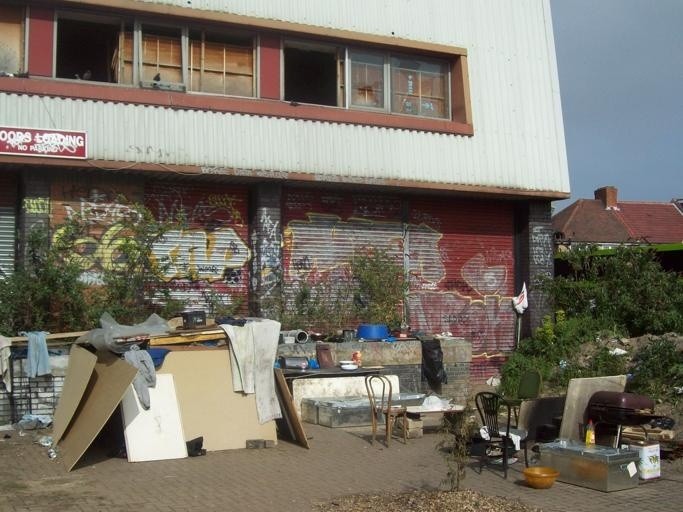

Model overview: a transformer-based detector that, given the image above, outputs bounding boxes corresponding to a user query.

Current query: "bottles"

[352,350,362,367]
[585,420,596,448]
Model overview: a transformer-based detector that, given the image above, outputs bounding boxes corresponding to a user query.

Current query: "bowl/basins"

[523,467,560,489]
[357,324,388,339]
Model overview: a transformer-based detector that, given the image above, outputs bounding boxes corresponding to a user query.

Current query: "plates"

[339,361,358,371]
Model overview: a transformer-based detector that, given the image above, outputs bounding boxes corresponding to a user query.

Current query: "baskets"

[175,310,206,329]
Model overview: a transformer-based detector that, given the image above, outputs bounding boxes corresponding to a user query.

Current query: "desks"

[404,405,477,440]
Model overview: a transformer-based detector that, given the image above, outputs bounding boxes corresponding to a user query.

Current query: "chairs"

[473,366,542,481]
[364,372,409,447]
